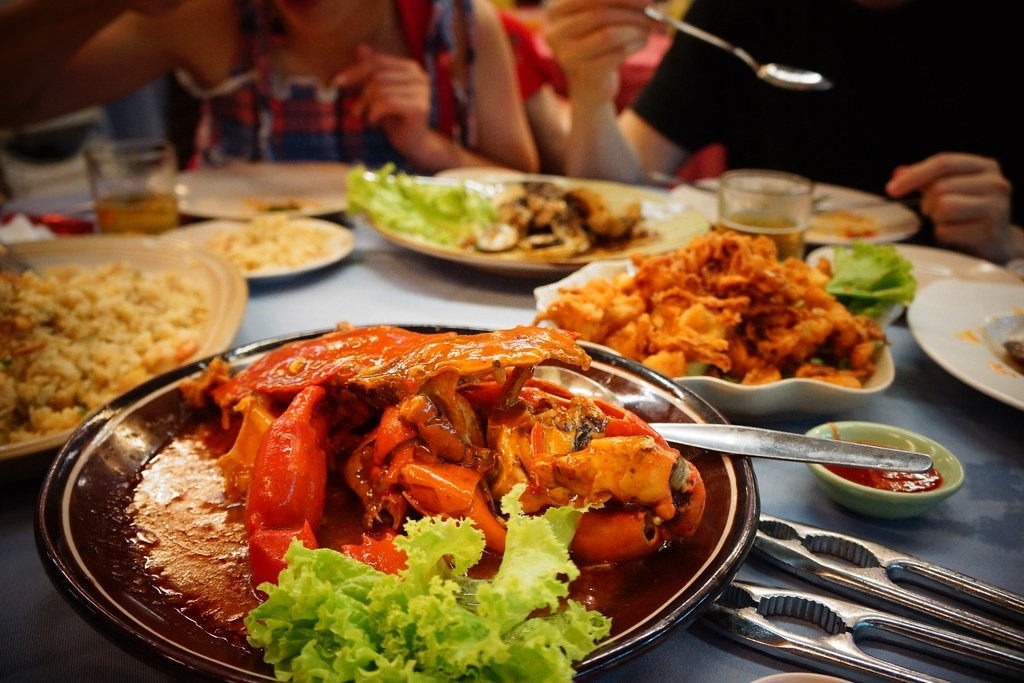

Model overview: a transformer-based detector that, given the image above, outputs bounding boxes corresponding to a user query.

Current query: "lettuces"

[822,235,918,323]
[341,158,495,252]
[242,481,612,683]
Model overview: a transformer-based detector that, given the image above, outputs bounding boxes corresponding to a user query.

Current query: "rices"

[1,257,210,450]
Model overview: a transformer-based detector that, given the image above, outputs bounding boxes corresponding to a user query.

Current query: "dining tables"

[1,178,1024,683]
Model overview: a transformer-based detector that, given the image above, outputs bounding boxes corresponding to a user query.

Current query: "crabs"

[178,324,706,603]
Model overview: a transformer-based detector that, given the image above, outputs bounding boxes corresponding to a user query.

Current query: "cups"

[83,137,179,237]
[716,169,813,263]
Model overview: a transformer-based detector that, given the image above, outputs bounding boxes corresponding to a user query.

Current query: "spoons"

[642,6,832,91]
[529,366,933,473]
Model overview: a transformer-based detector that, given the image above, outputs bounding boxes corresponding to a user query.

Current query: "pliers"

[700,509,1023,682]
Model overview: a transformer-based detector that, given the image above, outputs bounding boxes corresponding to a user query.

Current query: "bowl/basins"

[805,421,965,520]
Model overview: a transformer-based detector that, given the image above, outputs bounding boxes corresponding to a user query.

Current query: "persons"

[553,0,1024,263]
[1,0,541,178]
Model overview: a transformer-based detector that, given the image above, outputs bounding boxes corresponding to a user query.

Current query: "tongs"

[698,581,1024,683]
[752,512,1024,652]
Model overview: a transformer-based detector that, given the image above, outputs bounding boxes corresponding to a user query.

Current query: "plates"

[168,161,352,218]
[533,260,895,418]
[154,216,356,281]
[33,324,761,682]
[672,177,922,250]
[905,279,1024,410]
[1,236,248,461]
[365,172,714,279]
[805,243,1000,306]
